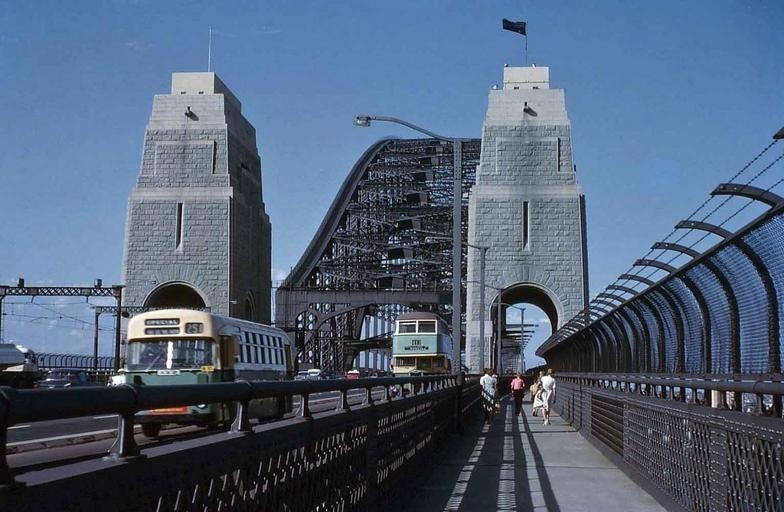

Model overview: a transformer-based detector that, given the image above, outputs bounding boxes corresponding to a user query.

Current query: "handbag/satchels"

[494,402,501,414]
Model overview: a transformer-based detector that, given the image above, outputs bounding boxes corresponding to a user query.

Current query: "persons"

[529,379,539,417]
[540,368,556,427]
[486,369,498,418]
[479,367,498,425]
[509,373,525,416]
[534,370,547,422]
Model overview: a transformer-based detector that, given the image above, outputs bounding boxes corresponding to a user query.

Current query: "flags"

[502,17,527,36]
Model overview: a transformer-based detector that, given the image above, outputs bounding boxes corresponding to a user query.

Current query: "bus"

[126,308,296,439]
[389,310,454,380]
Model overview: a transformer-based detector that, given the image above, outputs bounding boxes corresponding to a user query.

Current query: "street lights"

[422,234,530,394]
[352,114,465,378]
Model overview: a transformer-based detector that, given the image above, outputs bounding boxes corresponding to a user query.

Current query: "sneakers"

[544,421,551,425]
[532,410,538,416]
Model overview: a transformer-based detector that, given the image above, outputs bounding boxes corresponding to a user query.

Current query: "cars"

[295,365,388,382]
[0,341,127,390]
